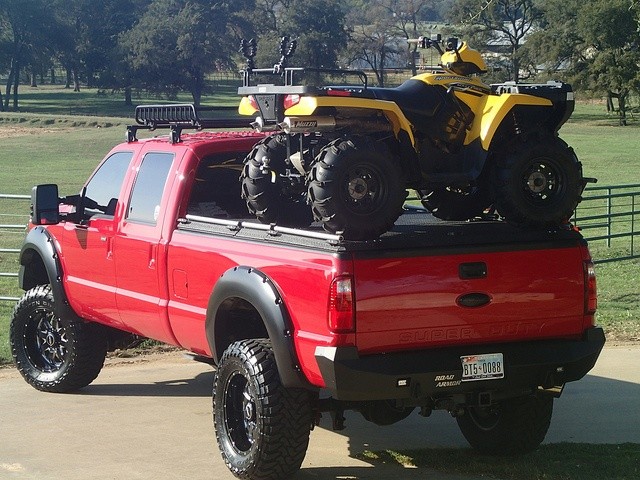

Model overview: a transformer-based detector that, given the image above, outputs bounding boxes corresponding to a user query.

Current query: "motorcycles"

[234,30,587,237]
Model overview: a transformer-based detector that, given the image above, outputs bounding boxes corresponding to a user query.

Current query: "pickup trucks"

[8,102,606,480]
